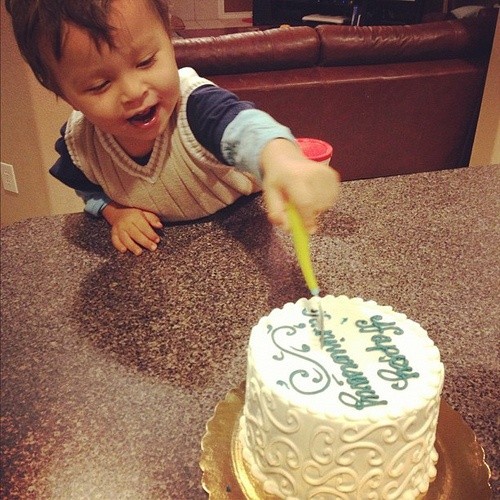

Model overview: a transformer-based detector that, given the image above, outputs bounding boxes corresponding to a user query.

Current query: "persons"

[4,0,343,257]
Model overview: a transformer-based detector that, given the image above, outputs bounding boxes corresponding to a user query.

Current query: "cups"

[294,138,334,169]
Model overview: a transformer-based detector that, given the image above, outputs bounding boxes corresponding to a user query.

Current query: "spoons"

[285,201,325,346]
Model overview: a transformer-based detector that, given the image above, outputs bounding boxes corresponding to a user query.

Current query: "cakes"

[237,294,446,500]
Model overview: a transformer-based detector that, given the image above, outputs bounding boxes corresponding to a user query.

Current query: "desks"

[0,164,500,500]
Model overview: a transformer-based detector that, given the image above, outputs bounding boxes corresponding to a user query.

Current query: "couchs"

[173,17,497,182]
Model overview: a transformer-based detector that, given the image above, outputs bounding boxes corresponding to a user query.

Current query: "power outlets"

[0,163,18,193]
